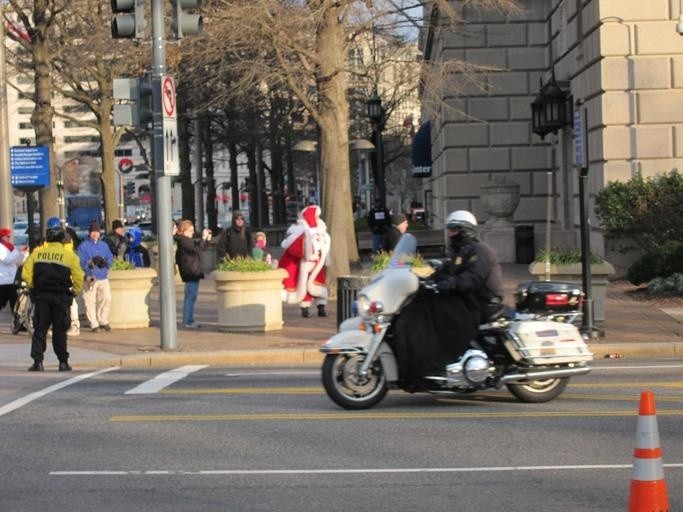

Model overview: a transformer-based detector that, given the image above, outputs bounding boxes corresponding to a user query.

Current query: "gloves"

[88,256,106,269]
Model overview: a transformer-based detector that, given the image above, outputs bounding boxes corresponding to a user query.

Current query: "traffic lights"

[109,0,147,42]
[112,78,152,131]
[169,0,203,41]
[127,182,135,194]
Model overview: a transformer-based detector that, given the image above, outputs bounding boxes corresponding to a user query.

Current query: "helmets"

[447,210,480,242]
[47,217,60,228]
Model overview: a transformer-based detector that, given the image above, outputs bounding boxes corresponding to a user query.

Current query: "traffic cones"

[623,390,672,512]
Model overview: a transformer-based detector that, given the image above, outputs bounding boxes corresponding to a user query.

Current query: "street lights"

[367,84,389,250]
[56,156,81,232]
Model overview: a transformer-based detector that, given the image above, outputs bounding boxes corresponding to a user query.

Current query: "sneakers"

[184,321,201,328]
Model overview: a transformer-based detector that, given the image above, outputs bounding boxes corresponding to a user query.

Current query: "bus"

[62,196,101,228]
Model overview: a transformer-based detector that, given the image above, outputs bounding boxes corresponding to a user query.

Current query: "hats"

[112,220,123,229]
[89,219,100,232]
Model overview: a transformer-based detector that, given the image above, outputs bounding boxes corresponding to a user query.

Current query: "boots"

[301,307,312,318]
[66,324,80,336]
[28,359,43,371]
[317,304,329,316]
[59,359,72,371]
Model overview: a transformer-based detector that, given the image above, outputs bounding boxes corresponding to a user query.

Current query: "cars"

[137,222,151,236]
[123,207,149,227]
[173,207,249,229]
[12,221,39,243]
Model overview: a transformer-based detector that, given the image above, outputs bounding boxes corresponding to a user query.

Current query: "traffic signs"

[571,101,586,171]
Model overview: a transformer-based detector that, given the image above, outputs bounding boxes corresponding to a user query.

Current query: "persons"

[278,205,331,317]
[173,220,213,329]
[156,220,178,278]
[217,210,250,265]
[352,196,409,253]
[422,210,504,321]
[250,232,271,267]
[0,216,151,371]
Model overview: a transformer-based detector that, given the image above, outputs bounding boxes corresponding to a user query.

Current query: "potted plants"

[360,250,435,284]
[527,246,616,324]
[211,254,289,334]
[150,238,177,286]
[105,255,159,332]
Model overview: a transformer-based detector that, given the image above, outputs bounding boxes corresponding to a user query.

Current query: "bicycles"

[9,280,34,335]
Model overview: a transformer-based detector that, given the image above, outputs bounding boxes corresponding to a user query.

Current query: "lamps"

[528,75,550,141]
[539,65,575,136]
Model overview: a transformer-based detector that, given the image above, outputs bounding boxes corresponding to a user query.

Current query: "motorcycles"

[318,232,595,411]
